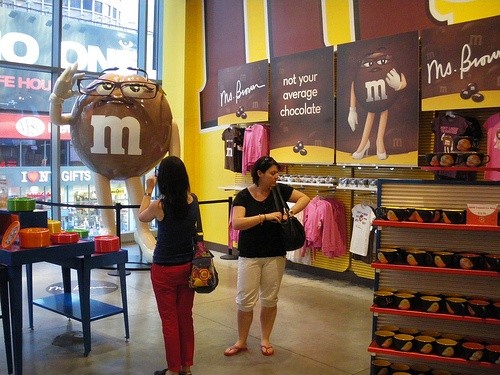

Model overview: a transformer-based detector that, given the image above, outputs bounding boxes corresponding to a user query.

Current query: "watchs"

[289,209,294,217]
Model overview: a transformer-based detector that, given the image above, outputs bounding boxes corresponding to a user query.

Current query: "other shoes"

[154,369,179,375]
[179,367,192,375]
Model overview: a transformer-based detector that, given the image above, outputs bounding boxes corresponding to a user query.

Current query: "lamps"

[9,9,86,33]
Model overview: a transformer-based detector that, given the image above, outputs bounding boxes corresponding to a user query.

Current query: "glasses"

[259,157,273,170]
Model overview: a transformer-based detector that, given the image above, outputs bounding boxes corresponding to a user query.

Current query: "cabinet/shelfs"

[368,217,500,375]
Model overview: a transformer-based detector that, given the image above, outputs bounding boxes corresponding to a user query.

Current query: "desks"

[0,239,130,375]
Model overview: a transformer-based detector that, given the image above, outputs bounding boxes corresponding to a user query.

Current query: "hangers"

[355,193,374,210]
[308,190,338,205]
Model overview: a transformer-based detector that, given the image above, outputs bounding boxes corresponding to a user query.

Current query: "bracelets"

[259,213,262,225]
[144,193,151,196]
[263,213,266,220]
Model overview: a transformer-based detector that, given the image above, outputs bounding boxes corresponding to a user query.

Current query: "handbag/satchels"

[272,183,305,251]
[189,193,218,293]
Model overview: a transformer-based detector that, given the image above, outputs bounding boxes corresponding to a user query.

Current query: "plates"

[1,197,121,254]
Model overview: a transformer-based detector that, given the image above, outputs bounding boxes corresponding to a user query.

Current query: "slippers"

[261,345,274,356]
[224,345,247,355]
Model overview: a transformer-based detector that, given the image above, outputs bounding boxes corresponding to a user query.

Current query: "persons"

[224,156,310,356]
[137,155,199,375]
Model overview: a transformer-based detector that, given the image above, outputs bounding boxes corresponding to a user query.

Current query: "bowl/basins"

[373,289,500,320]
[373,325,500,365]
[377,246,500,272]
[370,358,500,375]
[374,206,466,224]
